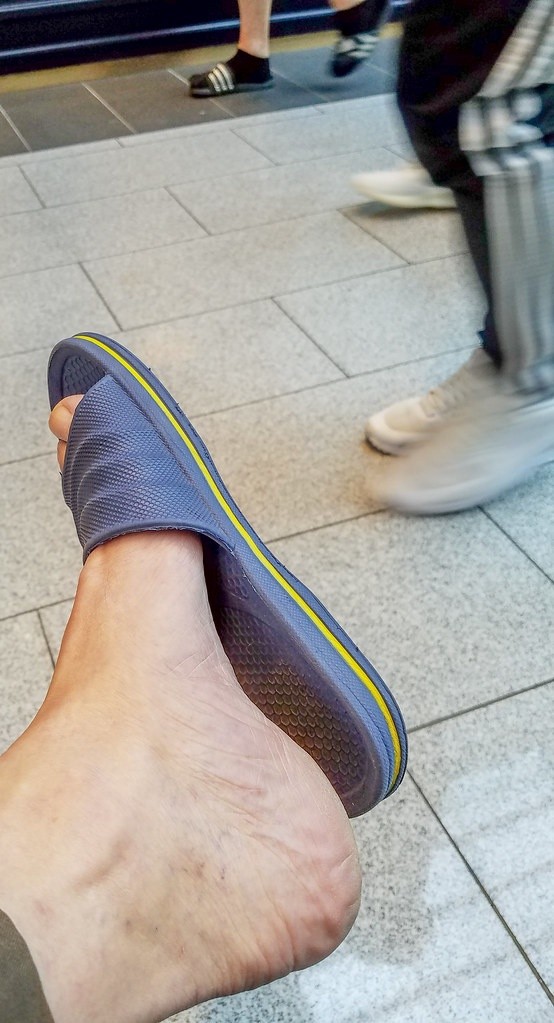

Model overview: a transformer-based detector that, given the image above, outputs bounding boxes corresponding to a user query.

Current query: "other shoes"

[359,170,458,209]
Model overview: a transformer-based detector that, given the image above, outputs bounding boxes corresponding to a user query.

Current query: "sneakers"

[363,394,554,513]
[365,349,497,454]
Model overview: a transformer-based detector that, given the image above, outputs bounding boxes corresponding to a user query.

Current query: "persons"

[181,0,554,516]
[1,333,409,1023]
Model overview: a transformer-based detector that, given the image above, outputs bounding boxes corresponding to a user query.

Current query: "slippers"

[46,333,404,818]
[330,0,394,78]
[191,63,275,96]
